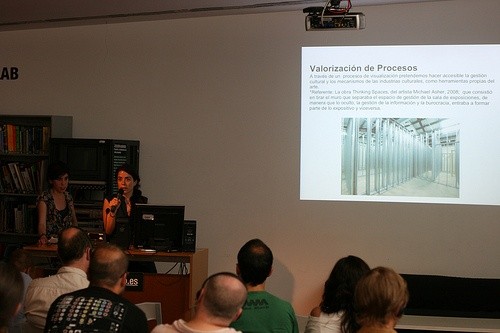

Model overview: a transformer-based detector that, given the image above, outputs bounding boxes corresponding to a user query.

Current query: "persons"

[44,243,149,333]
[305,255,370,333]
[355,266,408,333]
[0,236,34,333]
[149,272,247,333]
[38,161,78,244]
[24,226,92,333]
[228,239,300,333]
[103,167,158,274]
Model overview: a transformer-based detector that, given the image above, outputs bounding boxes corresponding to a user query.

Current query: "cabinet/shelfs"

[0,115,107,265]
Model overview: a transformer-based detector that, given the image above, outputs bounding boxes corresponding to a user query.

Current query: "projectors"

[304,13,366,31]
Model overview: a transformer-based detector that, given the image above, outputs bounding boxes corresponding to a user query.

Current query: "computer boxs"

[184,220,196,252]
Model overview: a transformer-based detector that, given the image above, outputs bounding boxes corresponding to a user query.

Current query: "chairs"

[135,302,162,325]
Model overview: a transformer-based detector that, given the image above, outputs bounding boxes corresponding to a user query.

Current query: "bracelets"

[40,233,46,237]
[110,213,116,218]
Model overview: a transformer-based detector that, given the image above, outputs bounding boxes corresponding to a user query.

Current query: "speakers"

[115,216,131,250]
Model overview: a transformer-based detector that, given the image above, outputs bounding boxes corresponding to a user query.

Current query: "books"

[0,160,47,194]
[0,201,38,234]
[0,124,48,154]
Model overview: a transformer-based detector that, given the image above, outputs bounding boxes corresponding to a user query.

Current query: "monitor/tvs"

[133,203,185,251]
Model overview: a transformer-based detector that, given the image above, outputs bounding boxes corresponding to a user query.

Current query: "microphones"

[111,188,124,213]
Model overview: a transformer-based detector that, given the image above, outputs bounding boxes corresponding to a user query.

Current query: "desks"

[21,245,209,325]
[393,315,500,333]
